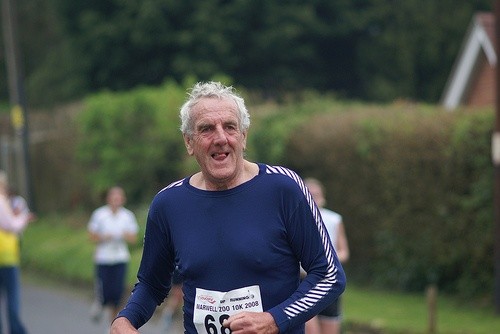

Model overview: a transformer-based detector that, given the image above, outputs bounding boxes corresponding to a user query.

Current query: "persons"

[300,177,350,334]
[0,170,30,334]
[88,186,139,322]
[109,81,346,334]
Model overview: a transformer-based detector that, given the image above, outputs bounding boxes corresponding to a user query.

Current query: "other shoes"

[160,309,173,332]
[89,302,103,324]
